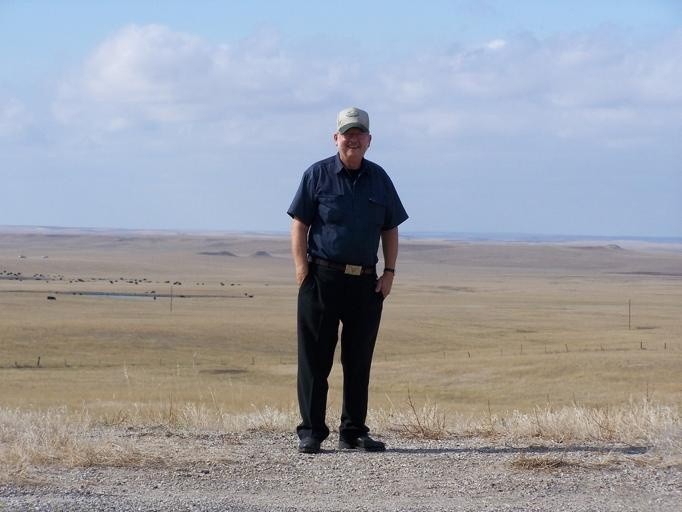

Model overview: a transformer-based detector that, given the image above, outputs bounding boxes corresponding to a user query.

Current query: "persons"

[287,107,408,453]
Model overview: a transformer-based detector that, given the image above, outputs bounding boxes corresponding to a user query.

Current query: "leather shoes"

[298,437,320,453]
[339,433,386,451]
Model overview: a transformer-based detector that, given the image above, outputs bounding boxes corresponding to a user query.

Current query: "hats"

[336,108,369,134]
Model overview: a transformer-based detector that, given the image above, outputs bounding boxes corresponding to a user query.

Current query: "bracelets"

[384,268,395,276]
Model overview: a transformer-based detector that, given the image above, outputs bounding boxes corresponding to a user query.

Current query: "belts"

[306,256,376,276]
